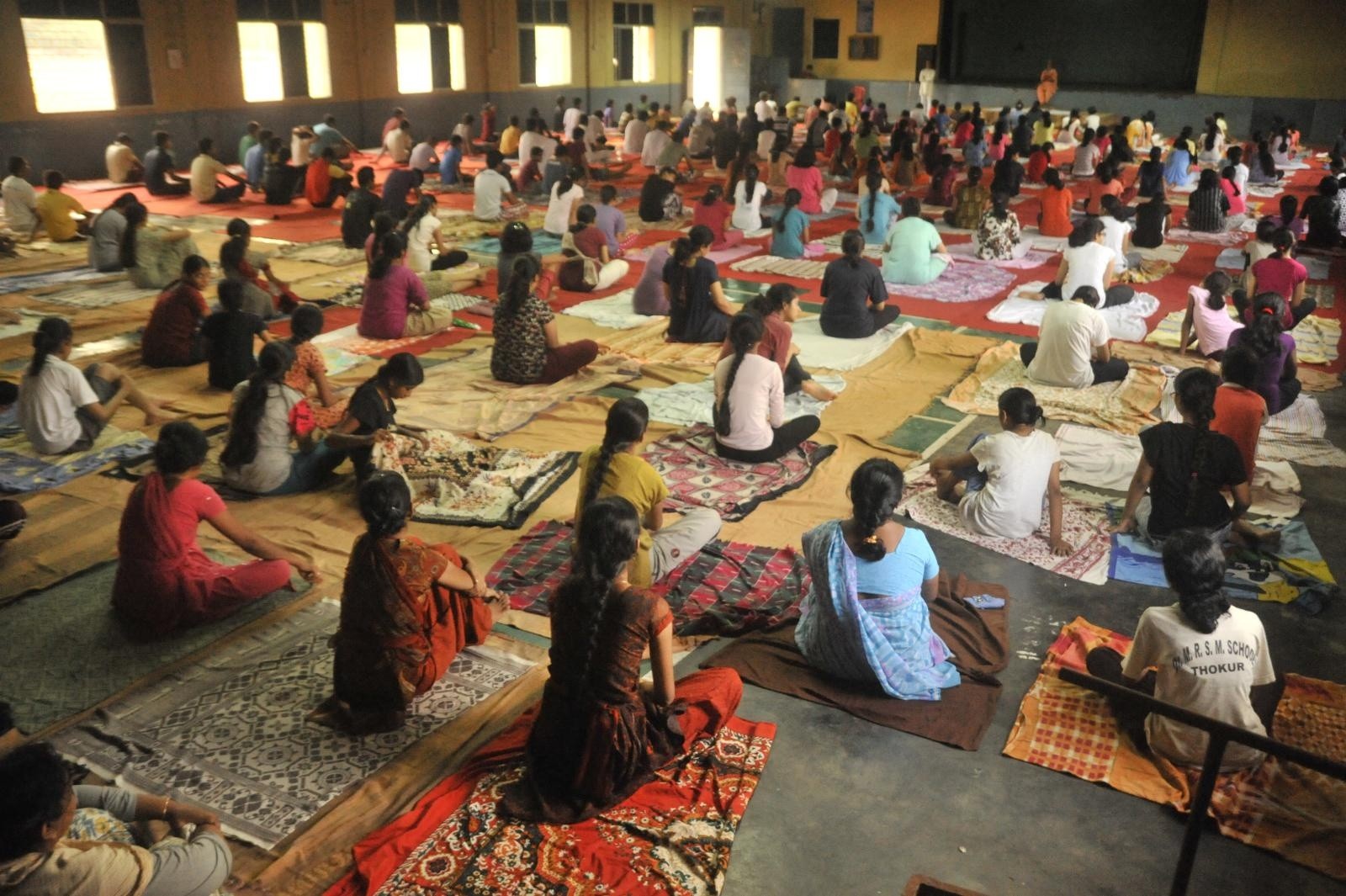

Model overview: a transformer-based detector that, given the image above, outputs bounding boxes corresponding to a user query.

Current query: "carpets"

[0,131,1346,896]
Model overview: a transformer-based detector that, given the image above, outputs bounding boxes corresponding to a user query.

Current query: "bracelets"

[163,795,173,823]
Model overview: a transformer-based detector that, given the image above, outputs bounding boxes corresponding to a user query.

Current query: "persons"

[1020,285,1130,390]
[16,316,186,454]
[928,389,1076,557]
[573,397,723,589]
[503,495,742,824]
[319,470,510,736]
[0,52,1345,391]
[0,743,233,896]
[330,352,431,478]
[490,255,599,387]
[663,225,740,344]
[218,342,348,497]
[710,311,820,466]
[1086,532,1287,772]
[1218,291,1302,418]
[819,231,899,339]
[110,420,323,630]
[1206,345,1269,488]
[356,231,453,341]
[792,456,963,703]
[140,253,218,368]
